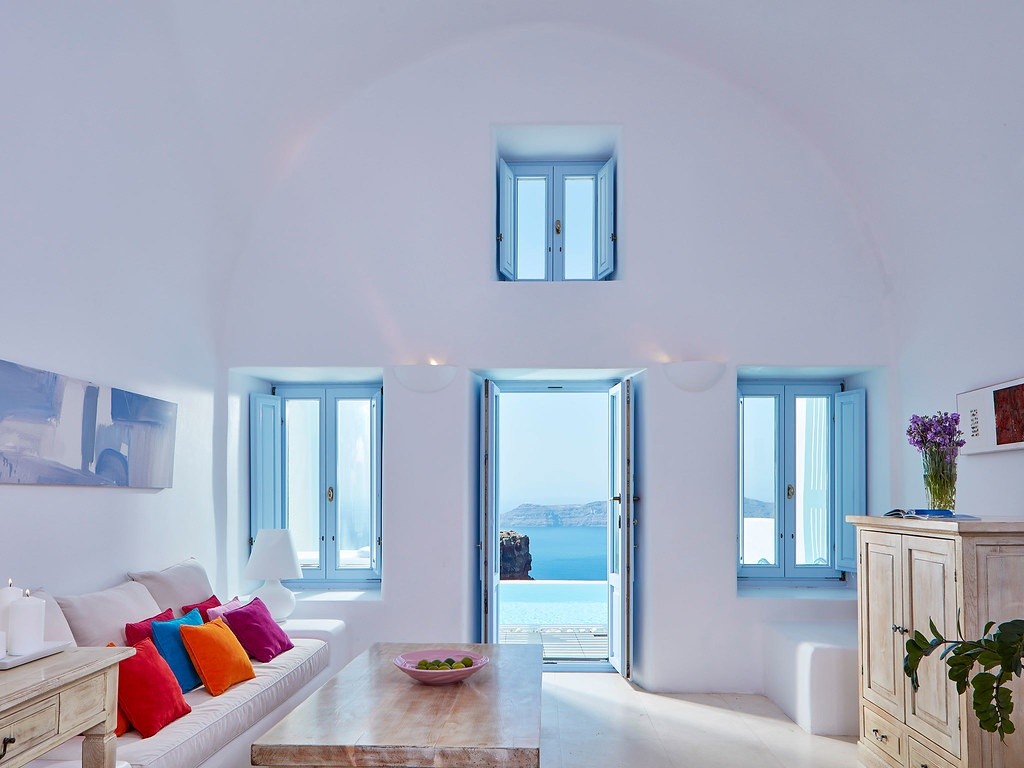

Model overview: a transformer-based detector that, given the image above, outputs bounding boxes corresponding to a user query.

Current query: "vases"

[920,446,956,515]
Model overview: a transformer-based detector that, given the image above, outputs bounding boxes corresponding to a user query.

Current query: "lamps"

[243,528,303,623]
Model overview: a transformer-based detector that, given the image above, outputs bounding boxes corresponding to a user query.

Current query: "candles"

[8,590,45,656]
[0,579,23,632]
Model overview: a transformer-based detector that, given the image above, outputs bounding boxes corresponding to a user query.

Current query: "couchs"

[24,556,332,768]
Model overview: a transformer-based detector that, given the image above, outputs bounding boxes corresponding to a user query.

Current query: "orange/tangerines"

[415,657,474,670]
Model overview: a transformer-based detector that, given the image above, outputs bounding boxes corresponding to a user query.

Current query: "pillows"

[23,587,77,647]
[99,641,129,739]
[150,609,203,693]
[181,595,222,622]
[52,581,159,649]
[223,597,295,661]
[208,597,245,627]
[181,617,257,696]
[110,639,191,740]
[127,557,218,616]
[124,605,173,648]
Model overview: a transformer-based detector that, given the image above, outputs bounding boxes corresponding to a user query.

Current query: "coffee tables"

[249,639,546,768]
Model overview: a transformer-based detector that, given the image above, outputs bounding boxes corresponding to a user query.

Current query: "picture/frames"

[956,377,1024,456]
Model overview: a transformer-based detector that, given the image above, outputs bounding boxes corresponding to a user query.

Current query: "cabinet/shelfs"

[846,512,1024,768]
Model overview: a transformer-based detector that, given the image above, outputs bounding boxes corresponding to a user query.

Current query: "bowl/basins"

[393,649,490,684]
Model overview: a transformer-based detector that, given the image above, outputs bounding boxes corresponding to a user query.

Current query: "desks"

[0,645,137,768]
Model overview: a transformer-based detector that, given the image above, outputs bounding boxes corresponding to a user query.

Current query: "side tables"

[284,616,346,663]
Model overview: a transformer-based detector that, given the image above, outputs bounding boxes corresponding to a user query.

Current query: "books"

[884,509,980,520]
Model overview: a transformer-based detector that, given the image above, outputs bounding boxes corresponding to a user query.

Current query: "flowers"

[906,411,965,450]
